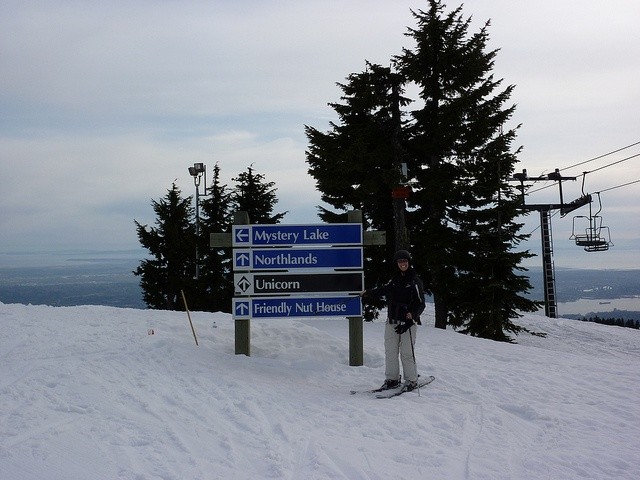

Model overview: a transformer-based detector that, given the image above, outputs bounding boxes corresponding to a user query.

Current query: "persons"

[360,250,425,392]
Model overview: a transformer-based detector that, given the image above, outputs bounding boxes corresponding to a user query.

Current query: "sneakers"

[385,379,398,388]
[401,380,417,392]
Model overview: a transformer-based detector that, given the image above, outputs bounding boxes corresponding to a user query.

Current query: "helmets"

[394,250,410,259]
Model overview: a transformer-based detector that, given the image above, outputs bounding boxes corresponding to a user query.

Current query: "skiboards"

[350,376,435,399]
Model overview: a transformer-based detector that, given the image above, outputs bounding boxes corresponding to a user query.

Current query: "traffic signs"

[231,271,364,295]
[233,249,364,271]
[231,224,366,247]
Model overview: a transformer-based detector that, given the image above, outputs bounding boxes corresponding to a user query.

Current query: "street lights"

[230,298,362,320]
[187,162,207,281]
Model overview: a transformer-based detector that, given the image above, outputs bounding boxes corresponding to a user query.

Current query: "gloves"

[394,318,414,334]
